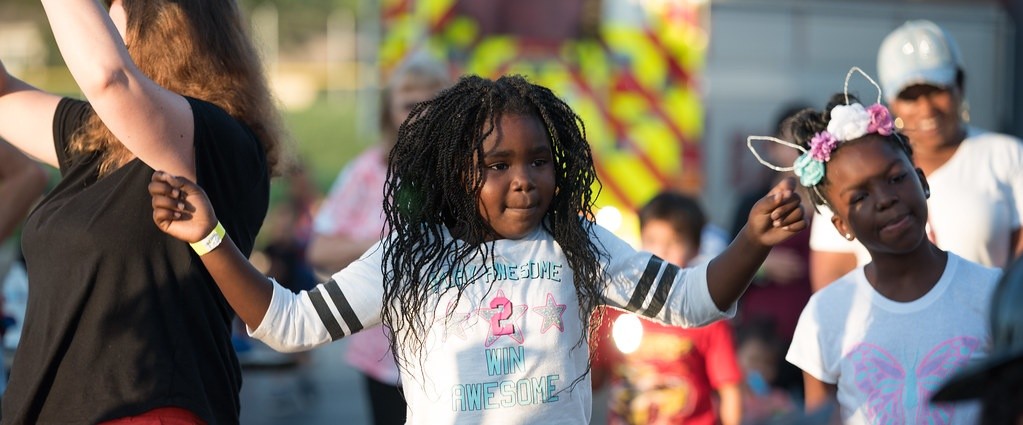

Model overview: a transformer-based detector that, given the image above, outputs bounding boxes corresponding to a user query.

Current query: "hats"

[876,19,959,102]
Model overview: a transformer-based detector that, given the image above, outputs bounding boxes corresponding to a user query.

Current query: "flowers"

[793,100,894,187]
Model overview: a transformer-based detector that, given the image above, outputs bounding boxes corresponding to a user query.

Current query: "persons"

[0,1,284,425]
[233,53,450,425]
[785,93,1004,425]
[808,17,1023,300]
[147,75,804,425]
[727,102,816,425]
[591,191,747,425]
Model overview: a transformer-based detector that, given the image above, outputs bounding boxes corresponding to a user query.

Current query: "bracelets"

[189,221,226,256]
